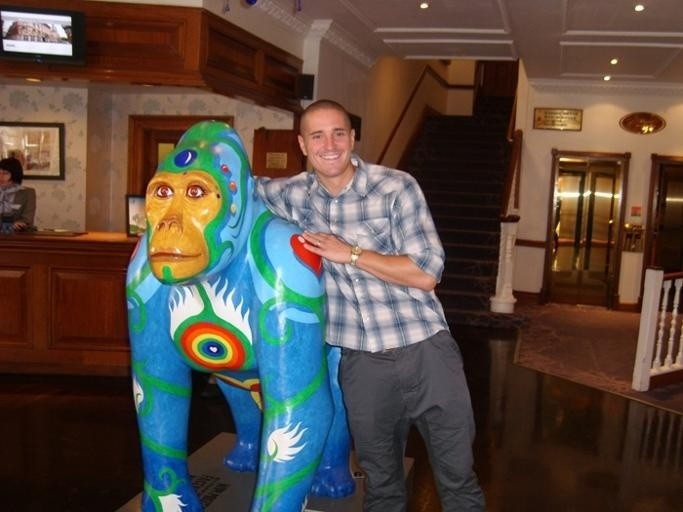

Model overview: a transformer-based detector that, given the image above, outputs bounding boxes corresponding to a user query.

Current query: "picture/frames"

[0,120,66,182]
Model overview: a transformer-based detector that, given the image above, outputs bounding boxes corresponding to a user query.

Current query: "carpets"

[512,301,683,416]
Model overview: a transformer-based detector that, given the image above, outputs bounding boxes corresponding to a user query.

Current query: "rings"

[317,240,322,248]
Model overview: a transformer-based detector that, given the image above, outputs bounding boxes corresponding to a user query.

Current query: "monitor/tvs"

[0,9,85,64]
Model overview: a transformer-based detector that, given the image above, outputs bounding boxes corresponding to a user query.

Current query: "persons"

[0,157,36,235]
[249,98,488,511]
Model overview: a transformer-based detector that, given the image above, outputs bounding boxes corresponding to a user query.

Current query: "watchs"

[348,244,362,267]
